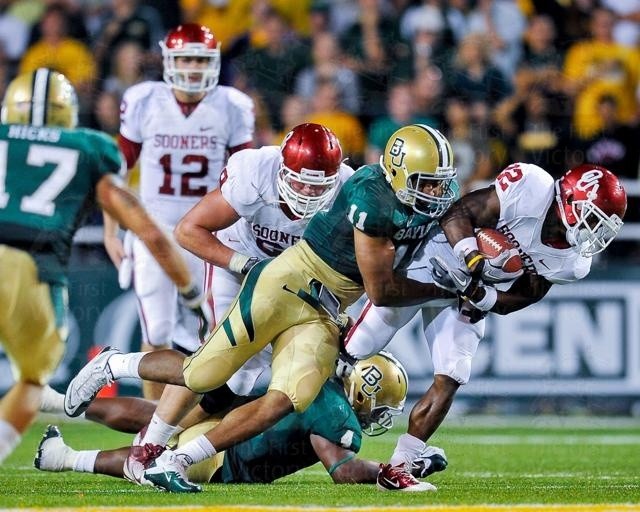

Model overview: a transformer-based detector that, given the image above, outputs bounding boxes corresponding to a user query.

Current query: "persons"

[0,1,166,131]
[64,122,460,494]
[102,19,257,405]
[1,67,209,466]
[33,343,448,482]
[172,119,358,393]
[333,160,629,476]
[181,1,638,186]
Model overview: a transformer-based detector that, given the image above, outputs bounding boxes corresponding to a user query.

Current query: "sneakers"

[121,424,201,491]
[378,464,438,493]
[32,424,68,472]
[64,346,123,417]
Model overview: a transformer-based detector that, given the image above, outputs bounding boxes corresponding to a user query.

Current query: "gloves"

[182,286,215,345]
[429,257,479,301]
[412,446,449,478]
[470,249,524,283]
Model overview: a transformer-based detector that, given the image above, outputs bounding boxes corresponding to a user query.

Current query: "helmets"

[554,164,628,258]
[161,23,221,95]
[0,68,78,127]
[344,350,408,436]
[379,124,459,218]
[277,123,344,219]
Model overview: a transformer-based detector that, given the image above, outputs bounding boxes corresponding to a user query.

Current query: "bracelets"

[225,252,251,271]
[472,284,497,316]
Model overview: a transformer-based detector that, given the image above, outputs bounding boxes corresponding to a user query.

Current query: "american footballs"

[474,227,521,275]
